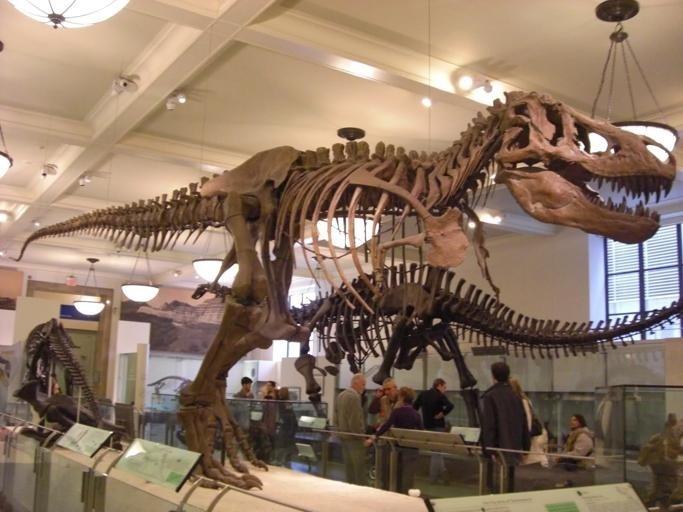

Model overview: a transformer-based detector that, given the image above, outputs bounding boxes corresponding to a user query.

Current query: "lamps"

[117,237,163,305]
[5,0,133,33]
[313,212,382,253]
[572,0,679,167]
[0,117,15,182]
[190,215,242,292]
[71,257,109,316]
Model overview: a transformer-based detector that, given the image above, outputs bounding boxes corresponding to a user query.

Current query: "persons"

[363,387,421,497]
[554,411,594,488]
[259,380,276,441]
[502,378,550,468]
[369,377,403,492]
[231,376,257,432]
[334,373,368,487]
[477,362,531,494]
[413,376,454,485]
[273,386,296,469]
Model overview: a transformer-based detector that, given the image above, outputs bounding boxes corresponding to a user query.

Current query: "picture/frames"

[284,385,301,408]
[312,373,324,396]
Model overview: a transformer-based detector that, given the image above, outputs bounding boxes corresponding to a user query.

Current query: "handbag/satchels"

[529,416,543,437]
